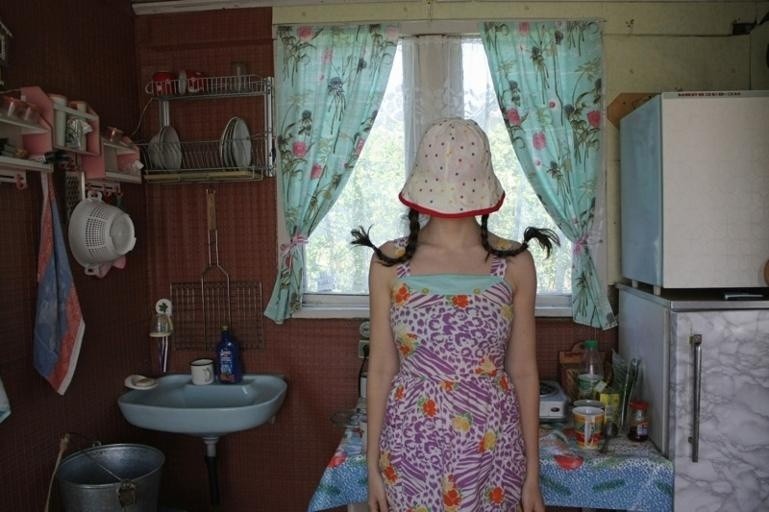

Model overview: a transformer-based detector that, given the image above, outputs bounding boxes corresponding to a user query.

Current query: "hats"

[399,120,505,217]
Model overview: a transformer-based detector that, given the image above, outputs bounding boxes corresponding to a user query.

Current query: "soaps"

[136,378,156,386]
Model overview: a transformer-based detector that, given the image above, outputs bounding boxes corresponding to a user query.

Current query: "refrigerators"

[614,280,769,512]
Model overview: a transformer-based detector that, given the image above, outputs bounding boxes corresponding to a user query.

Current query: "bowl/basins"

[146,126,183,173]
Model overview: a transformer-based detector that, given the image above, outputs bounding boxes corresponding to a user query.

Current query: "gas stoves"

[537,379,569,425]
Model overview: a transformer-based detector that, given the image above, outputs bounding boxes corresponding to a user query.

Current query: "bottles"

[230,61,248,93]
[0,94,42,124]
[627,400,650,442]
[572,340,606,399]
[106,127,124,145]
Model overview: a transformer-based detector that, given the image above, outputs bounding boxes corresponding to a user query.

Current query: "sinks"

[116,372,288,435]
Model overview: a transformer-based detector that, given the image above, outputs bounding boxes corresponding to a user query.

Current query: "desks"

[305,396,676,511]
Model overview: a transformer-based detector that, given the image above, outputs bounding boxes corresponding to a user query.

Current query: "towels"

[30,164,86,397]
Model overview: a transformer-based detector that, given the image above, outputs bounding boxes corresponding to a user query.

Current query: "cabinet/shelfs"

[137,73,277,185]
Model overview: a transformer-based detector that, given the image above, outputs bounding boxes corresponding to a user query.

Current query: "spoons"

[598,421,618,453]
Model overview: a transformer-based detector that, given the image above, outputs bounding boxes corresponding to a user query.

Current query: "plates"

[218,115,253,172]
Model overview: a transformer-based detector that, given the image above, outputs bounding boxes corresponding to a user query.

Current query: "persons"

[351,117,562,512]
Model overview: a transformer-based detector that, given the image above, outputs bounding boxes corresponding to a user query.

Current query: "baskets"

[68,191,136,275]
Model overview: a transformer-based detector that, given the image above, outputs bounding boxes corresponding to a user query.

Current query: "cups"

[189,357,216,386]
[359,372,368,398]
[68,98,89,152]
[149,313,174,335]
[358,419,368,452]
[46,89,67,148]
[571,400,609,453]
[144,69,208,97]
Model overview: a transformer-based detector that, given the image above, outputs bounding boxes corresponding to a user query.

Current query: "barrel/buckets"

[54,432,166,512]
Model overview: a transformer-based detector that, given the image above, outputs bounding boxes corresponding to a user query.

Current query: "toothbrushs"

[162,322,170,373]
[159,319,165,375]
